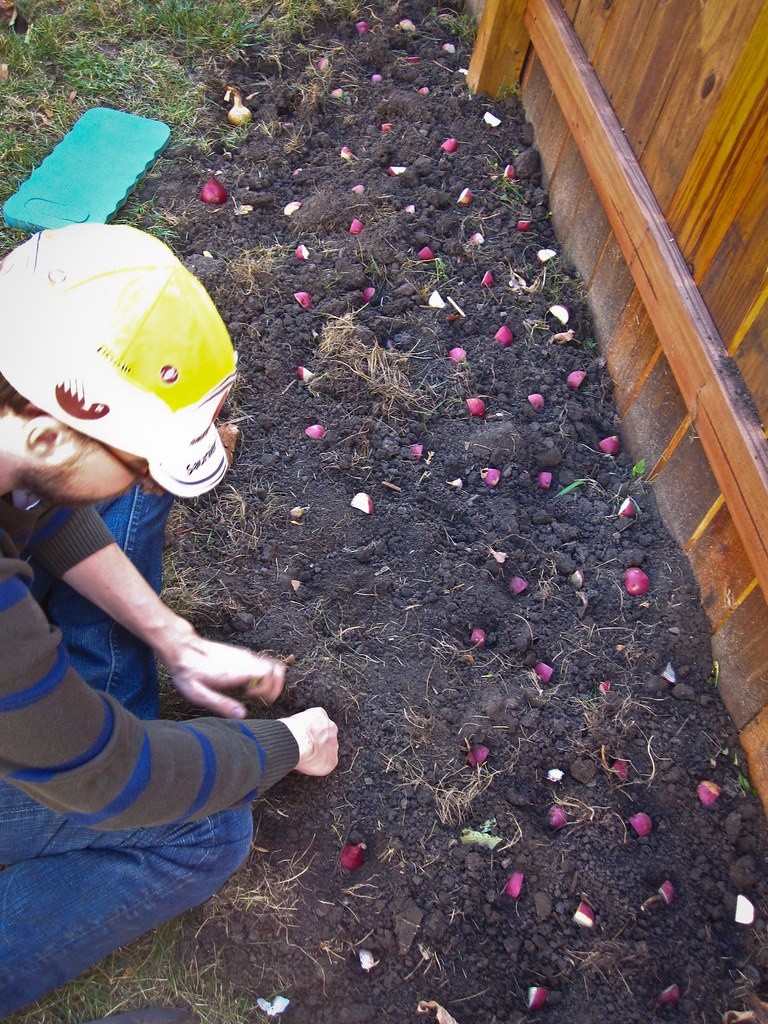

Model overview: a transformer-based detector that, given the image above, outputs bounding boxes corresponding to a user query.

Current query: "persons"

[0,226,340,1018]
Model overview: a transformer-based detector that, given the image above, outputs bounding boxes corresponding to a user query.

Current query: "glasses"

[92,435,167,497]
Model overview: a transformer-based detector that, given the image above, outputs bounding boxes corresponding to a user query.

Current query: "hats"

[0,223,237,497]
